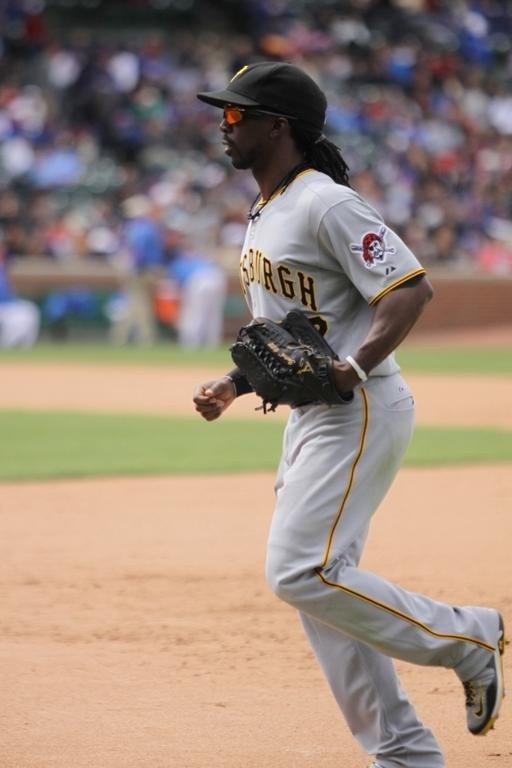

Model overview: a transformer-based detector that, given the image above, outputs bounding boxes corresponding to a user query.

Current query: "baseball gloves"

[230,307,355,406]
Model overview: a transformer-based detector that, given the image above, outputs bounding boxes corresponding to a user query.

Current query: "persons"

[3,0,510,352]
[193,60,505,768]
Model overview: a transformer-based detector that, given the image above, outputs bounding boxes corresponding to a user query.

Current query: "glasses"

[222,105,298,125]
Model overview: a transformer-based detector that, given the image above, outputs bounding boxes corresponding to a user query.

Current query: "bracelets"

[345,352,369,383]
[224,365,253,399]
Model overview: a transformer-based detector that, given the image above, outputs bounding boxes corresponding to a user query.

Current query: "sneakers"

[461,609,504,736]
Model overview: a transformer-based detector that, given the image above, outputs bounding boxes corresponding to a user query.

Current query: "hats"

[197,62,328,133]
